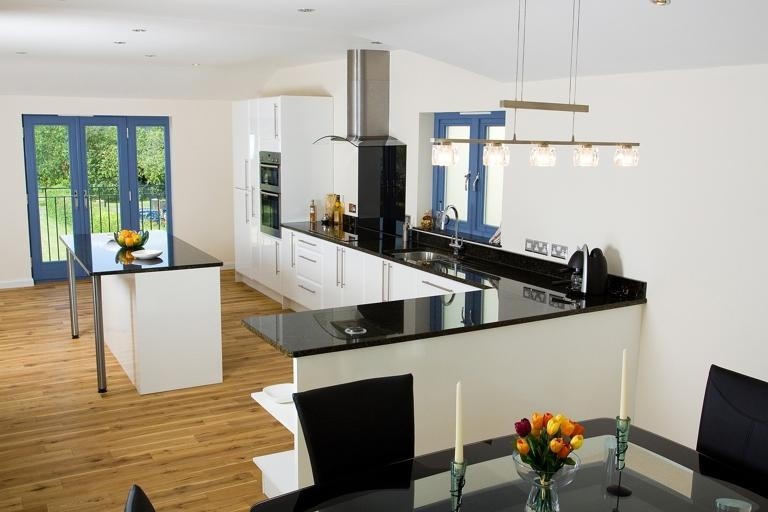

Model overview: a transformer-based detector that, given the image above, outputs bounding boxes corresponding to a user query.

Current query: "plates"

[130,257,163,265]
[130,249,164,259]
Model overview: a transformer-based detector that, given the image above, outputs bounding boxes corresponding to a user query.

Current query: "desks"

[60,229,223,394]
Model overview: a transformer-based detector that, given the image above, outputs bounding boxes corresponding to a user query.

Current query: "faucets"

[439,205,464,254]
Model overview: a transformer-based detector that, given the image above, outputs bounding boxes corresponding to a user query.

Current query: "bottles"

[333,195,343,237]
[435,201,445,232]
[310,200,316,222]
[403,224,409,241]
[422,210,432,231]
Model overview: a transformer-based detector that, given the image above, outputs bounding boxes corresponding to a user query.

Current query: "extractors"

[312,49,408,147]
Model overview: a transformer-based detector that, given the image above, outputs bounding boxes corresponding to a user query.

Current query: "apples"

[131,232,136,238]
[119,235,128,245]
[121,230,130,238]
[125,238,134,247]
[132,234,142,245]
[129,230,135,235]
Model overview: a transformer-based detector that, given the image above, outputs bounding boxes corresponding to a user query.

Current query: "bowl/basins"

[114,229,150,249]
[115,247,145,266]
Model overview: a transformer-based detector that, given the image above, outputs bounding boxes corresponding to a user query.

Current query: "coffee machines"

[565,243,607,300]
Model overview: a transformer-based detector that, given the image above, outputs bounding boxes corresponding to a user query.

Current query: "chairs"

[691,364,768,477]
[291,374,414,490]
[125,484,156,512]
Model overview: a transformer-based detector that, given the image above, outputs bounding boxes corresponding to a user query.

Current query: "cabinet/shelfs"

[241,220,650,501]
[232,96,333,309]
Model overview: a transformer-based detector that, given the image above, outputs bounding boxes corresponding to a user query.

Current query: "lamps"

[430,0,641,171]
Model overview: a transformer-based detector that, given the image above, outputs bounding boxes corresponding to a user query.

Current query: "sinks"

[389,250,457,265]
